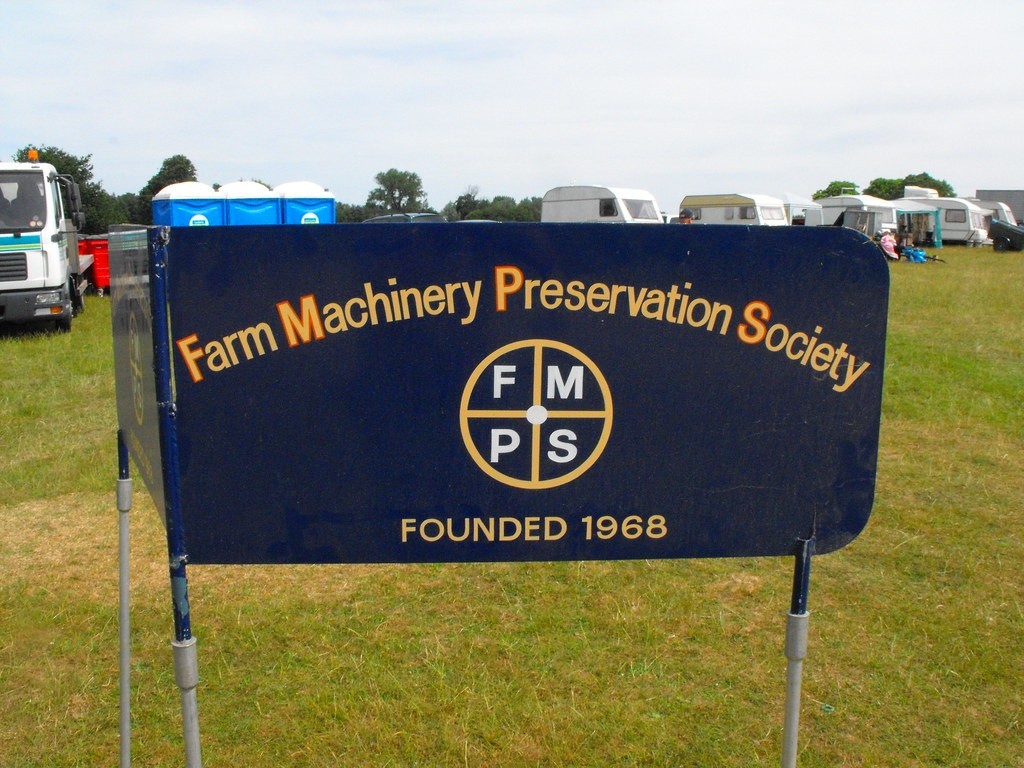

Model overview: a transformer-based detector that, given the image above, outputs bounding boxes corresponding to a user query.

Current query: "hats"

[679,209,693,219]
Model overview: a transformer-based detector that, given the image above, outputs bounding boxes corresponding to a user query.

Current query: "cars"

[360,211,448,223]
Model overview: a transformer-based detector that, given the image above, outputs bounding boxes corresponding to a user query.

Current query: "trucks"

[0,150,94,332]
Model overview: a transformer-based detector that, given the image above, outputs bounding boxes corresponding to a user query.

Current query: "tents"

[894,199,944,250]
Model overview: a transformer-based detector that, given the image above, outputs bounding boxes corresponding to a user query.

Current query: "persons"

[679,209,695,224]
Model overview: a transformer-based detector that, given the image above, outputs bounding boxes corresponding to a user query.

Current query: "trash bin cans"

[77,233,89,255]
[86,234,111,298]
